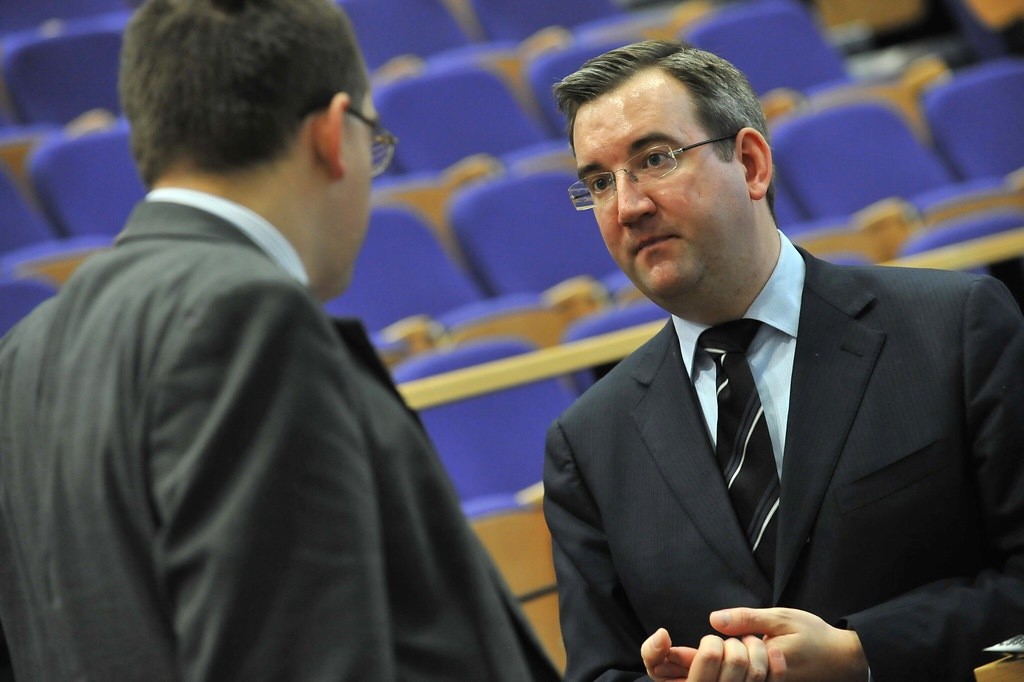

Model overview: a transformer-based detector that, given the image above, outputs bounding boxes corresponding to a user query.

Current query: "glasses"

[567,128,734,212]
[297,98,399,178]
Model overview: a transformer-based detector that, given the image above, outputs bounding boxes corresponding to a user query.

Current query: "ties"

[696,319,781,586]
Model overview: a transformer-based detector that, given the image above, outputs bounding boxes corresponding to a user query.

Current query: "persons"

[0,0,564,682]
[543,37,1024,682]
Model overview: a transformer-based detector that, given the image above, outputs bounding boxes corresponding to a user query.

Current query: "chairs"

[0,0,1024,604]
